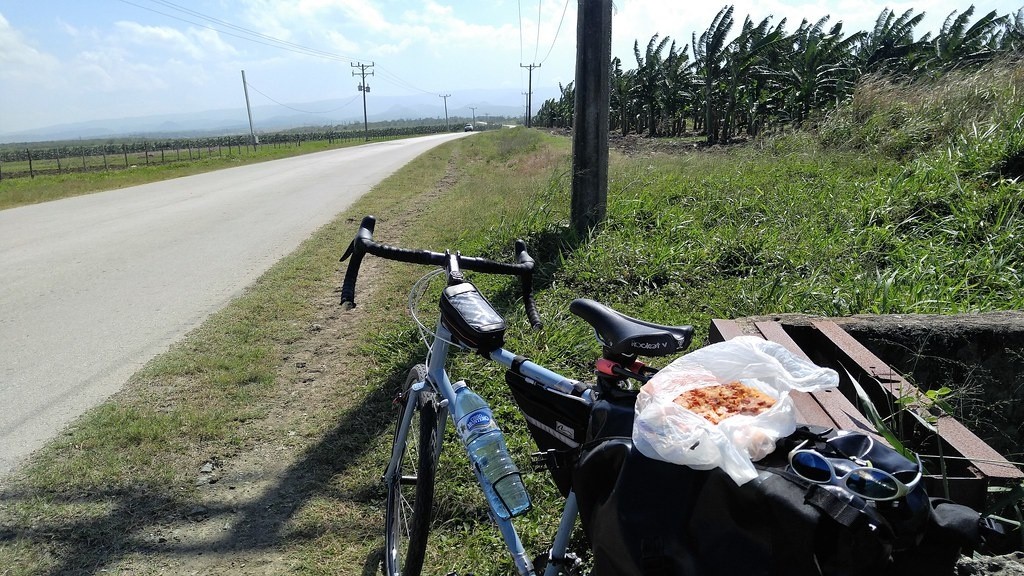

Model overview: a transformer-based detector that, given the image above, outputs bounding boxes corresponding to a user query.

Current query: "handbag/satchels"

[530,436,981,576]
[632,335,839,486]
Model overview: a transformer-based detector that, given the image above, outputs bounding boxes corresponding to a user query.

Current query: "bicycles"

[340,215,917,576]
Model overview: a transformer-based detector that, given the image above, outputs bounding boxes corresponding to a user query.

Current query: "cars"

[464,123,474,131]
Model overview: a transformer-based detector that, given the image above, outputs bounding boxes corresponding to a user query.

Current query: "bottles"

[451,379,531,518]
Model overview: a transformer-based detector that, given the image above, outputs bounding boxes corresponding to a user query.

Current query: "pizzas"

[669,382,777,425]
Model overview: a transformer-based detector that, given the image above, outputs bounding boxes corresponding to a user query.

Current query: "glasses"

[788,439,922,501]
